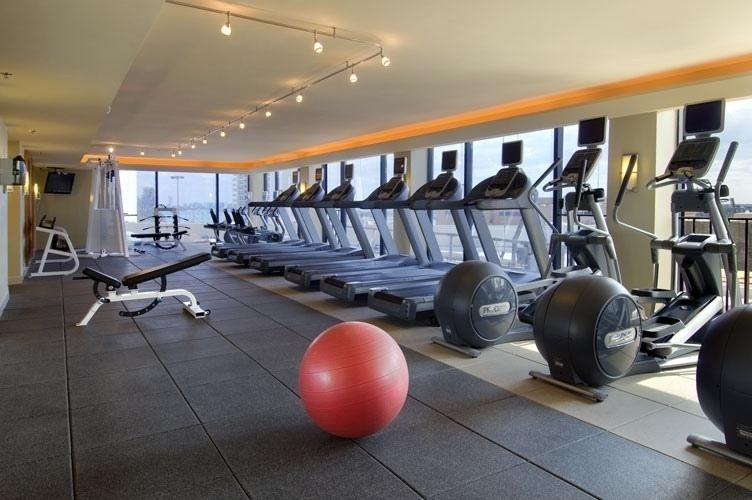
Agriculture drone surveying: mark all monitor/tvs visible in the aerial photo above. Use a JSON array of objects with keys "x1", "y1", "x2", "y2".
[
  {"x1": 248, "y1": 191, "x2": 252, "y2": 202},
  {"x1": 393, "y1": 156, "x2": 408, "y2": 175},
  {"x1": 683, "y1": 97, "x2": 726, "y2": 136},
  {"x1": 344, "y1": 164, "x2": 355, "y2": 179},
  {"x1": 44, "y1": 172, "x2": 76, "y2": 194},
  {"x1": 501, "y1": 140, "x2": 523, "y2": 166},
  {"x1": 272, "y1": 191, "x2": 278, "y2": 200},
  {"x1": 315, "y1": 168, "x2": 324, "y2": 183},
  {"x1": 292, "y1": 171, "x2": 300, "y2": 184},
  {"x1": 261, "y1": 191, "x2": 268, "y2": 200},
  {"x1": 441, "y1": 150, "x2": 458, "y2": 172},
  {"x1": 577, "y1": 116, "x2": 608, "y2": 147}
]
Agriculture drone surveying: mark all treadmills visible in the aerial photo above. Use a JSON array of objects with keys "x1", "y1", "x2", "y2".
[
  {"x1": 319, "y1": 149, "x2": 481, "y2": 307},
  {"x1": 283, "y1": 156, "x2": 432, "y2": 294},
  {"x1": 366, "y1": 140, "x2": 553, "y2": 328},
  {"x1": 211, "y1": 163, "x2": 375, "y2": 275}
]
